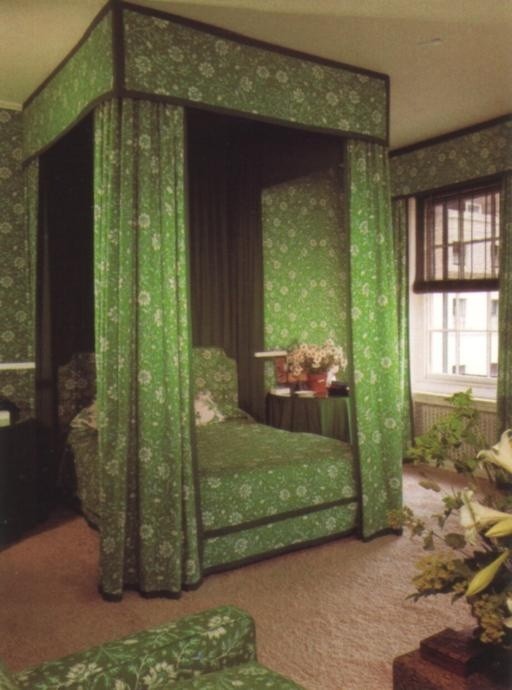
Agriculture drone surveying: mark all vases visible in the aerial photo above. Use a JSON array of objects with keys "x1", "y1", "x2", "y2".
[{"x1": 307, "y1": 369, "x2": 329, "y2": 391}]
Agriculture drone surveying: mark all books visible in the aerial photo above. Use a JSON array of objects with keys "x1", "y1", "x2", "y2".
[{"x1": 266, "y1": 380, "x2": 350, "y2": 401}]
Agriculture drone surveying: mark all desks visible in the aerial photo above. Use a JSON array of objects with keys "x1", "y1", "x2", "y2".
[{"x1": 268, "y1": 386, "x2": 353, "y2": 444}]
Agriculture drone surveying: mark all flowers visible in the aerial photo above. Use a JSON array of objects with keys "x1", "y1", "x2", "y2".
[
  {"x1": 379, "y1": 388, "x2": 511, "y2": 651},
  {"x1": 290, "y1": 337, "x2": 347, "y2": 383}
]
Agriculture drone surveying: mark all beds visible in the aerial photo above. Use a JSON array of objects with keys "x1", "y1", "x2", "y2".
[{"x1": 59, "y1": 345, "x2": 359, "y2": 573}]
